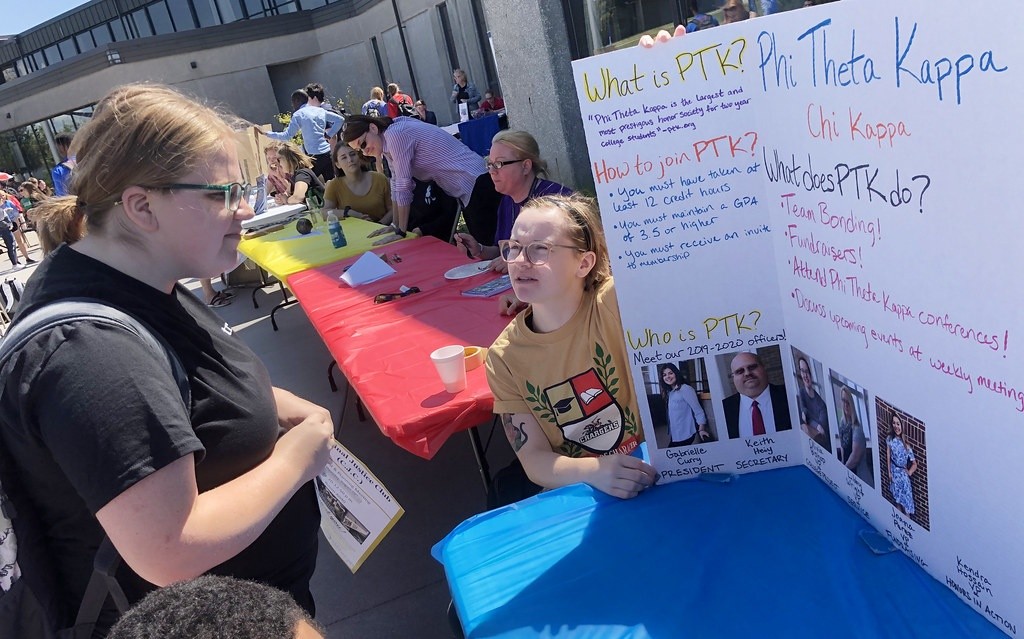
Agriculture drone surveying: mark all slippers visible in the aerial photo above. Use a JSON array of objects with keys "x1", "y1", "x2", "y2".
[
  {"x1": 206, "y1": 294, "x2": 231, "y2": 308},
  {"x1": 216, "y1": 291, "x2": 236, "y2": 299}
]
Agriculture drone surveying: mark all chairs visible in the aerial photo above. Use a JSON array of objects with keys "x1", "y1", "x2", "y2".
[{"x1": 458, "y1": 114, "x2": 501, "y2": 157}]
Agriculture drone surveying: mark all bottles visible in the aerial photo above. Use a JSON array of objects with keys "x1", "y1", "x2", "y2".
[{"x1": 326, "y1": 210, "x2": 347, "y2": 249}]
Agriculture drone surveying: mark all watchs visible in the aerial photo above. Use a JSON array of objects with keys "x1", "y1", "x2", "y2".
[{"x1": 395, "y1": 228, "x2": 407, "y2": 238}]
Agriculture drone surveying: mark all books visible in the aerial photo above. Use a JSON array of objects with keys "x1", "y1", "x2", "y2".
[{"x1": 461, "y1": 275, "x2": 512, "y2": 298}]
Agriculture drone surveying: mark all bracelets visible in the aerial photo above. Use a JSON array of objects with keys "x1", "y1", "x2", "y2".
[
  {"x1": 391, "y1": 223, "x2": 397, "y2": 229},
  {"x1": 343, "y1": 206, "x2": 351, "y2": 217},
  {"x1": 698, "y1": 430, "x2": 704, "y2": 432},
  {"x1": 473, "y1": 243, "x2": 483, "y2": 257},
  {"x1": 286, "y1": 196, "x2": 290, "y2": 204}
]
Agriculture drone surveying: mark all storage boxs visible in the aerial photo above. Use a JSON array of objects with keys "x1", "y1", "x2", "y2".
[{"x1": 227, "y1": 258, "x2": 267, "y2": 285}]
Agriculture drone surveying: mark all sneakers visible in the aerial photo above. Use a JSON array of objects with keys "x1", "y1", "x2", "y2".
[
  {"x1": 25, "y1": 259, "x2": 42, "y2": 268},
  {"x1": 12, "y1": 261, "x2": 26, "y2": 272}
]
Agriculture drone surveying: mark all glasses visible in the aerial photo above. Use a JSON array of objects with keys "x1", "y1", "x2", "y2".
[
  {"x1": 453, "y1": 75, "x2": 461, "y2": 79},
  {"x1": 415, "y1": 104, "x2": 423, "y2": 108},
  {"x1": 484, "y1": 159, "x2": 524, "y2": 170},
  {"x1": 114, "y1": 181, "x2": 251, "y2": 212},
  {"x1": 277, "y1": 157, "x2": 282, "y2": 163},
  {"x1": 19, "y1": 189, "x2": 24, "y2": 193},
  {"x1": 729, "y1": 362, "x2": 761, "y2": 375},
  {"x1": 486, "y1": 96, "x2": 493, "y2": 100},
  {"x1": 498, "y1": 240, "x2": 587, "y2": 266},
  {"x1": 356, "y1": 132, "x2": 367, "y2": 157},
  {"x1": 841, "y1": 398, "x2": 850, "y2": 404},
  {"x1": 799, "y1": 368, "x2": 810, "y2": 373}
]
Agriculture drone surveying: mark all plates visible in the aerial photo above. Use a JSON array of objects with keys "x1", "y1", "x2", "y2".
[{"x1": 444, "y1": 259, "x2": 493, "y2": 280}]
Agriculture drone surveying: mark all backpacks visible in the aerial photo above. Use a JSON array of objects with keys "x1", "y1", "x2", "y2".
[
  {"x1": 366, "y1": 101, "x2": 381, "y2": 119},
  {"x1": 1, "y1": 300, "x2": 192, "y2": 639},
  {"x1": 390, "y1": 94, "x2": 421, "y2": 122}
]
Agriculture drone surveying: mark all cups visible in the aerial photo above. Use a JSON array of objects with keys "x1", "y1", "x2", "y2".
[{"x1": 429, "y1": 344, "x2": 466, "y2": 394}]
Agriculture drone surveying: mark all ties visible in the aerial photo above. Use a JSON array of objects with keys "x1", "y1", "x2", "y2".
[{"x1": 751, "y1": 400, "x2": 766, "y2": 436}]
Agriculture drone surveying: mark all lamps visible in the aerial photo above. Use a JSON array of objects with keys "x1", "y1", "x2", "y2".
[
  {"x1": 106, "y1": 50, "x2": 122, "y2": 66},
  {"x1": 6, "y1": 112, "x2": 10, "y2": 118},
  {"x1": 190, "y1": 62, "x2": 197, "y2": 69}
]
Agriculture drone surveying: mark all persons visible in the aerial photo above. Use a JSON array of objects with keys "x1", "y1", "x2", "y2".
[
  {"x1": 200, "y1": 69, "x2": 573, "y2": 318},
  {"x1": 659, "y1": 362, "x2": 709, "y2": 445},
  {"x1": 686, "y1": 0, "x2": 815, "y2": 34},
  {"x1": 105, "y1": 576, "x2": 332, "y2": 638},
  {"x1": 722, "y1": 352, "x2": 792, "y2": 439},
  {"x1": 798, "y1": 357, "x2": 832, "y2": 454},
  {"x1": 0, "y1": 131, "x2": 77, "y2": 271},
  {"x1": 839, "y1": 386, "x2": 875, "y2": 488},
  {"x1": 886, "y1": 413, "x2": 917, "y2": 518},
  {"x1": 0, "y1": 83, "x2": 332, "y2": 639},
  {"x1": 485, "y1": 25, "x2": 685, "y2": 500}
]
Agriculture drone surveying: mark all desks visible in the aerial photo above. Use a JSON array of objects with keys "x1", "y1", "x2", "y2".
[
  {"x1": 441, "y1": 109, "x2": 506, "y2": 138},
  {"x1": 237, "y1": 212, "x2": 418, "y2": 329},
  {"x1": 428, "y1": 440, "x2": 1011, "y2": 638},
  {"x1": 288, "y1": 238, "x2": 530, "y2": 511}
]
